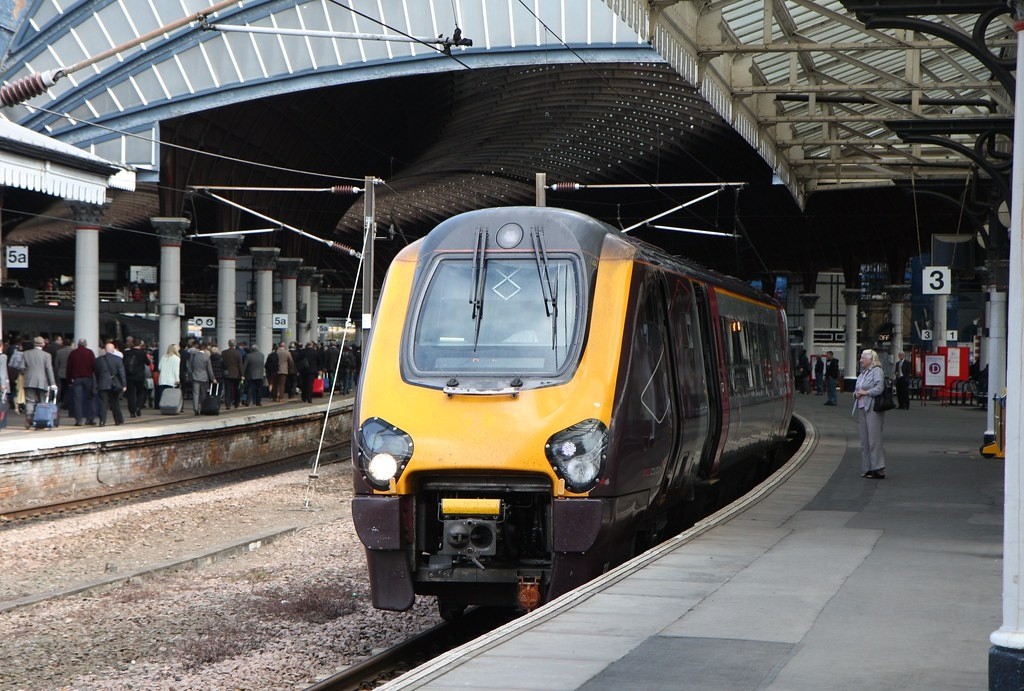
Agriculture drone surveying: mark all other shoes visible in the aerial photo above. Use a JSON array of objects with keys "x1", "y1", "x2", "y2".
[
  {"x1": 195, "y1": 412, "x2": 199, "y2": 416},
  {"x1": 116, "y1": 420, "x2": 123, "y2": 425},
  {"x1": 141, "y1": 383, "x2": 353, "y2": 410},
  {"x1": 100, "y1": 421, "x2": 104, "y2": 427},
  {"x1": 895, "y1": 405, "x2": 909, "y2": 410},
  {"x1": 800, "y1": 390, "x2": 825, "y2": 396},
  {"x1": 824, "y1": 400, "x2": 835, "y2": 405},
  {"x1": 130, "y1": 411, "x2": 141, "y2": 417},
  {"x1": 25, "y1": 416, "x2": 31, "y2": 430},
  {"x1": 75, "y1": 422, "x2": 81, "y2": 426},
  {"x1": 85, "y1": 421, "x2": 96, "y2": 425}
]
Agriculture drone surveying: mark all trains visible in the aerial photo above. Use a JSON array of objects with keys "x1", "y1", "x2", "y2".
[
  {"x1": 349, "y1": 205, "x2": 795, "y2": 621},
  {"x1": 0, "y1": 279, "x2": 158, "y2": 351}
]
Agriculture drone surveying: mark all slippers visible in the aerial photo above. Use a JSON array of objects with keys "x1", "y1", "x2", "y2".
[
  {"x1": 866, "y1": 472, "x2": 885, "y2": 479},
  {"x1": 860, "y1": 471, "x2": 872, "y2": 477}
]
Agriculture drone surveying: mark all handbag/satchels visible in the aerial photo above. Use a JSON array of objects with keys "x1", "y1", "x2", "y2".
[
  {"x1": 873, "y1": 379, "x2": 897, "y2": 411},
  {"x1": 111, "y1": 375, "x2": 123, "y2": 393}
]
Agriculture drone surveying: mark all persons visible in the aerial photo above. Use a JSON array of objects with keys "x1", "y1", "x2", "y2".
[
  {"x1": 507, "y1": 309, "x2": 568, "y2": 348},
  {"x1": 796, "y1": 350, "x2": 810, "y2": 396},
  {"x1": 815, "y1": 352, "x2": 825, "y2": 396},
  {"x1": 1, "y1": 266, "x2": 363, "y2": 431},
  {"x1": 851, "y1": 349, "x2": 886, "y2": 480},
  {"x1": 821, "y1": 350, "x2": 839, "y2": 407},
  {"x1": 895, "y1": 351, "x2": 912, "y2": 410}
]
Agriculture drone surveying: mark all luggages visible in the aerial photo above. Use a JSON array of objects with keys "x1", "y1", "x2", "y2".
[
  {"x1": 311, "y1": 374, "x2": 324, "y2": 397},
  {"x1": 32, "y1": 386, "x2": 58, "y2": 430},
  {"x1": 0, "y1": 388, "x2": 9, "y2": 428},
  {"x1": 159, "y1": 383, "x2": 183, "y2": 414},
  {"x1": 322, "y1": 372, "x2": 329, "y2": 391},
  {"x1": 201, "y1": 382, "x2": 221, "y2": 415}
]
